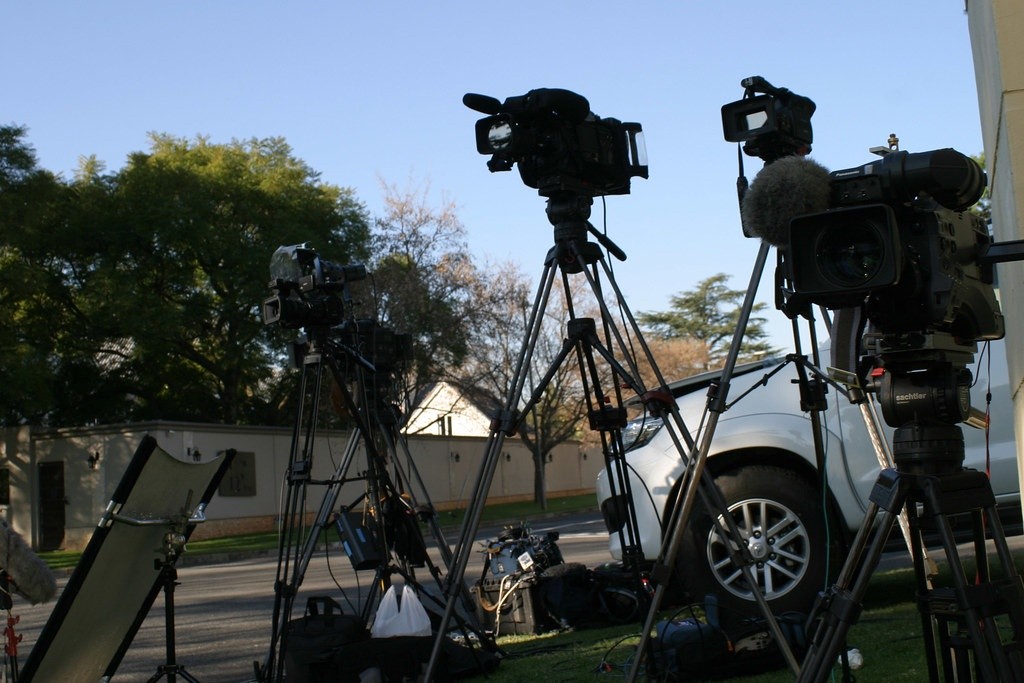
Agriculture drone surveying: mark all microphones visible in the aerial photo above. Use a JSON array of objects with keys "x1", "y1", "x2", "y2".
[
  {"x1": 463, "y1": 93, "x2": 505, "y2": 115},
  {"x1": 739, "y1": 154, "x2": 833, "y2": 250}
]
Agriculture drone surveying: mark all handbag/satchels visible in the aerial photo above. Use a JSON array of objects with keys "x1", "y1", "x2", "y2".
[
  {"x1": 371, "y1": 584, "x2": 433, "y2": 639},
  {"x1": 285, "y1": 595, "x2": 367, "y2": 680}
]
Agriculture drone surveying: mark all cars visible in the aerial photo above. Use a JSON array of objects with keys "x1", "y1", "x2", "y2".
[{"x1": 599, "y1": 226, "x2": 1019, "y2": 618}]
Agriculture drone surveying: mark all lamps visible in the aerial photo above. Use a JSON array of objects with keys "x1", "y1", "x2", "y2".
[
  {"x1": 503, "y1": 451, "x2": 511, "y2": 462},
  {"x1": 451, "y1": 451, "x2": 460, "y2": 462},
  {"x1": 187, "y1": 447, "x2": 201, "y2": 461},
  {"x1": 579, "y1": 451, "x2": 587, "y2": 461},
  {"x1": 546, "y1": 452, "x2": 553, "y2": 461},
  {"x1": 87, "y1": 451, "x2": 99, "y2": 468}
]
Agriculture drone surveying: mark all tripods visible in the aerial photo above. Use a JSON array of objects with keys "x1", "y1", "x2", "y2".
[{"x1": 262, "y1": 197, "x2": 1024, "y2": 682}]
